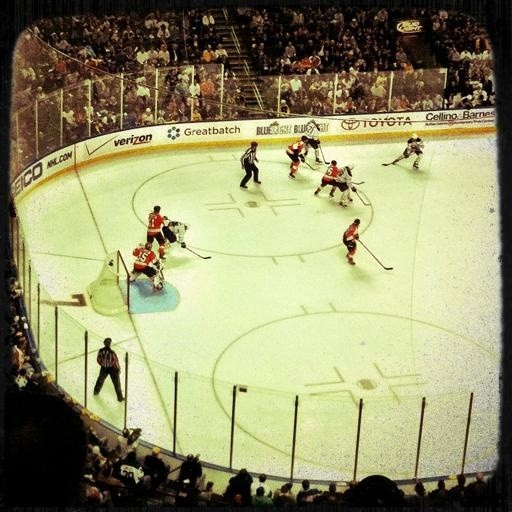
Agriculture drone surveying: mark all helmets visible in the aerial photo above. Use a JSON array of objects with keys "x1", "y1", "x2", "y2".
[
  {"x1": 251, "y1": 141, "x2": 258, "y2": 146},
  {"x1": 331, "y1": 160, "x2": 336, "y2": 165}
]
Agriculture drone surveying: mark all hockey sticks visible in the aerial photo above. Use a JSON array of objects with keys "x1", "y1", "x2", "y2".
[
  {"x1": 305, "y1": 162, "x2": 321, "y2": 171},
  {"x1": 356, "y1": 239, "x2": 394, "y2": 270},
  {"x1": 352, "y1": 182, "x2": 365, "y2": 185},
  {"x1": 356, "y1": 192, "x2": 372, "y2": 206},
  {"x1": 318, "y1": 144, "x2": 330, "y2": 164},
  {"x1": 177, "y1": 241, "x2": 212, "y2": 259},
  {"x1": 382, "y1": 152, "x2": 413, "y2": 166}
]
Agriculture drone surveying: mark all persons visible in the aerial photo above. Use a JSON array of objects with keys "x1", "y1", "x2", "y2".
[
  {"x1": 239, "y1": 141, "x2": 261, "y2": 189},
  {"x1": 342, "y1": 218, "x2": 360, "y2": 264},
  {"x1": 162, "y1": 221, "x2": 188, "y2": 249},
  {"x1": 146, "y1": 206, "x2": 166, "y2": 260},
  {"x1": 129, "y1": 243, "x2": 163, "y2": 290},
  {"x1": 337, "y1": 167, "x2": 357, "y2": 207},
  {"x1": 315, "y1": 159, "x2": 341, "y2": 197}
]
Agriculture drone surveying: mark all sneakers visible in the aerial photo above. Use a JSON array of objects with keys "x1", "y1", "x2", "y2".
[
  {"x1": 254, "y1": 180, "x2": 261, "y2": 184},
  {"x1": 289, "y1": 173, "x2": 295, "y2": 178},
  {"x1": 330, "y1": 193, "x2": 334, "y2": 197},
  {"x1": 315, "y1": 189, "x2": 319, "y2": 195},
  {"x1": 240, "y1": 184, "x2": 248, "y2": 189},
  {"x1": 315, "y1": 158, "x2": 322, "y2": 163}
]
